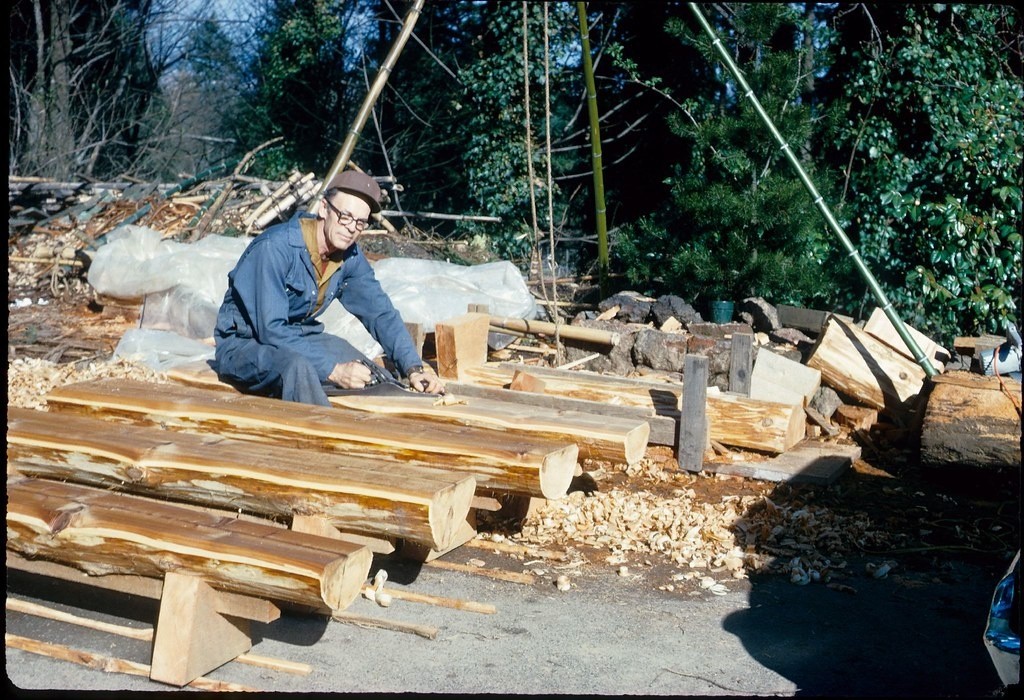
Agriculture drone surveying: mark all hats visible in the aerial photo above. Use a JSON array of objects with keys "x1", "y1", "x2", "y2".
[{"x1": 327, "y1": 170, "x2": 382, "y2": 213}]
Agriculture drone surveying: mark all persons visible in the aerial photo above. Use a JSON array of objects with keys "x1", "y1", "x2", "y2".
[{"x1": 214, "y1": 171, "x2": 444, "y2": 406}]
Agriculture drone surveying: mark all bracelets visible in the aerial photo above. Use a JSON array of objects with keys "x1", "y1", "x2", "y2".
[{"x1": 407, "y1": 366, "x2": 423, "y2": 379}]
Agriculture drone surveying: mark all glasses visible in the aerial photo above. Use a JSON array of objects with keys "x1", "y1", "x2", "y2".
[{"x1": 324, "y1": 196, "x2": 369, "y2": 231}]
